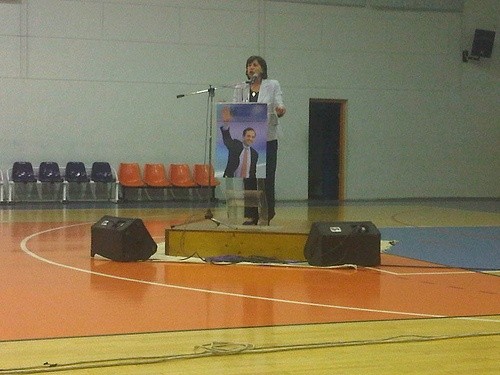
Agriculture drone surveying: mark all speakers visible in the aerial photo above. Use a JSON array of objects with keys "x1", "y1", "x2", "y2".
[
  {"x1": 91, "y1": 215, "x2": 158, "y2": 262},
  {"x1": 471, "y1": 29, "x2": 496, "y2": 58},
  {"x1": 303, "y1": 221, "x2": 381, "y2": 267}
]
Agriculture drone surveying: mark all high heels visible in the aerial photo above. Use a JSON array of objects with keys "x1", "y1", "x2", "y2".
[{"x1": 242, "y1": 216, "x2": 259, "y2": 225}]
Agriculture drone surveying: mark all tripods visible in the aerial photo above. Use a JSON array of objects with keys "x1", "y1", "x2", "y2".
[{"x1": 169, "y1": 81, "x2": 252, "y2": 229}]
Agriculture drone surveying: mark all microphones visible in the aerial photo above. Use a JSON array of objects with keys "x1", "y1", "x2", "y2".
[{"x1": 251, "y1": 74, "x2": 259, "y2": 84}]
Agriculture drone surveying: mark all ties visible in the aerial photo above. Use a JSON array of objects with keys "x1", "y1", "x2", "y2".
[{"x1": 239, "y1": 147, "x2": 249, "y2": 178}]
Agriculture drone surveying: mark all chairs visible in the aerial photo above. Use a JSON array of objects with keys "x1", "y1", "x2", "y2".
[{"x1": 6, "y1": 159, "x2": 220, "y2": 204}]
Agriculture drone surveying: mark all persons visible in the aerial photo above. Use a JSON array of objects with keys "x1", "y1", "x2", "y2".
[
  {"x1": 232, "y1": 56, "x2": 286, "y2": 225},
  {"x1": 220, "y1": 105, "x2": 259, "y2": 180}
]
